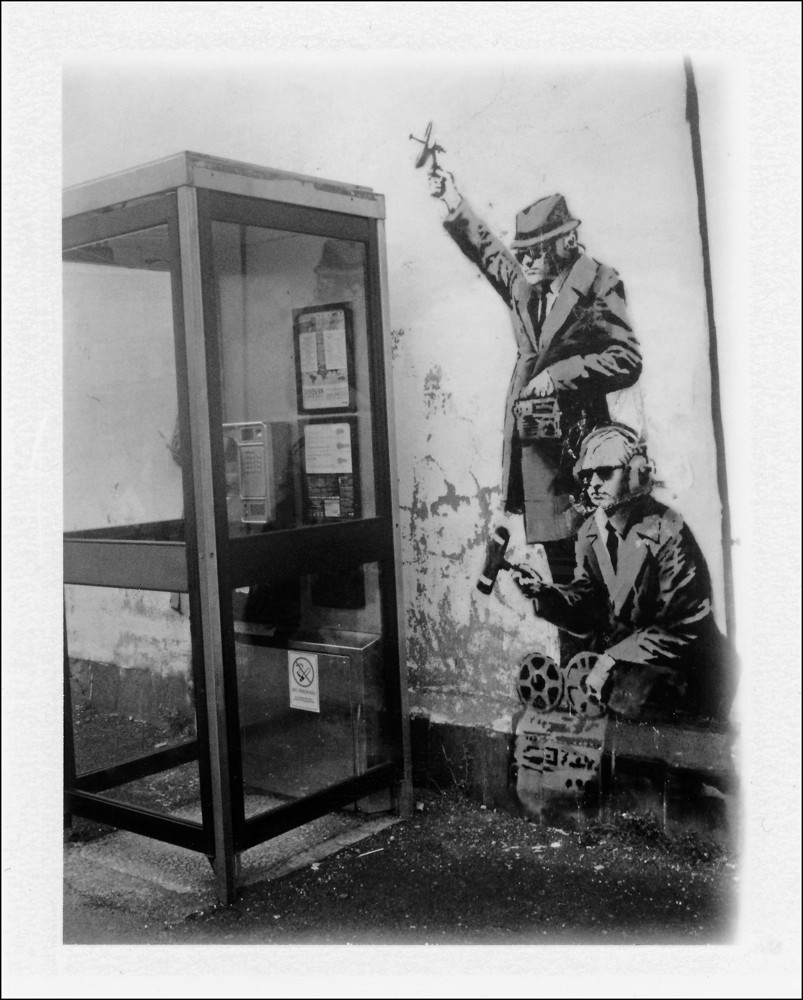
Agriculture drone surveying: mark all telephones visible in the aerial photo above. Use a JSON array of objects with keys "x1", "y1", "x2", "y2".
[{"x1": 221, "y1": 420, "x2": 290, "y2": 525}]
